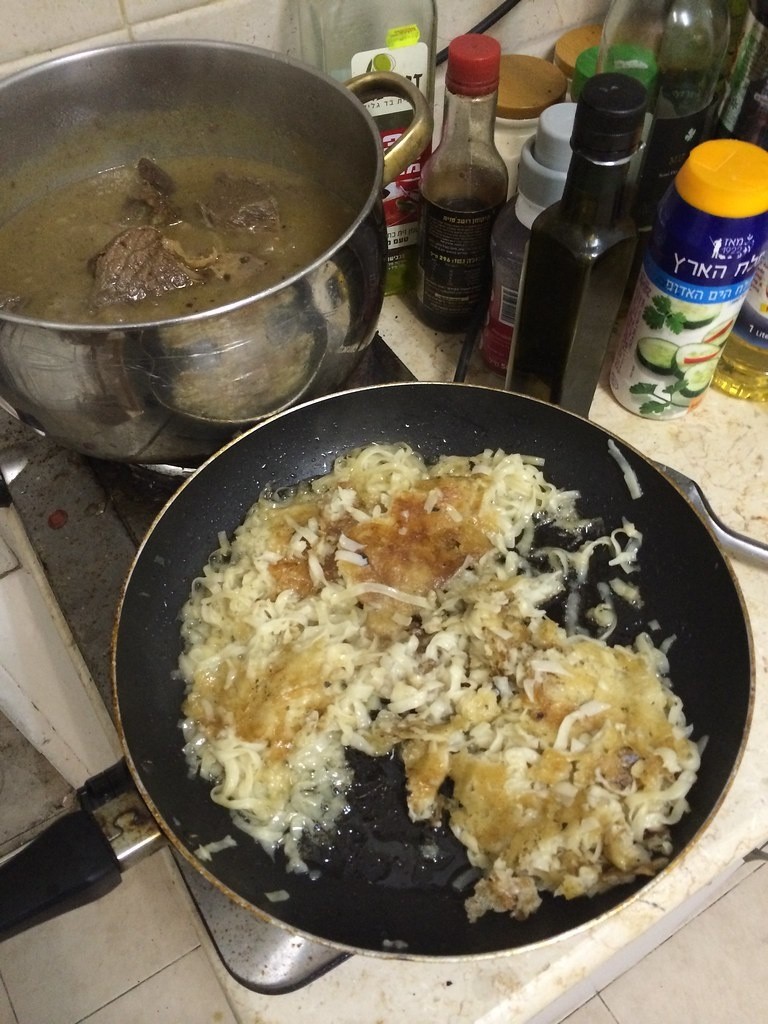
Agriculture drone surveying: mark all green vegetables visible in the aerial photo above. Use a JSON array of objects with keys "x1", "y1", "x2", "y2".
[
  {"x1": 629, "y1": 380, "x2": 690, "y2": 414},
  {"x1": 641, "y1": 295, "x2": 687, "y2": 336}
]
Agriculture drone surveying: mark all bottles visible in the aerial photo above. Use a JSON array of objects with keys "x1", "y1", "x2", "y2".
[
  {"x1": 595, "y1": 0, "x2": 731, "y2": 298},
  {"x1": 606, "y1": 138, "x2": 768, "y2": 420},
  {"x1": 417, "y1": 34, "x2": 508, "y2": 333},
  {"x1": 478, "y1": 102, "x2": 581, "y2": 377},
  {"x1": 295, "y1": 0, "x2": 437, "y2": 297},
  {"x1": 493, "y1": 24, "x2": 658, "y2": 200},
  {"x1": 713, "y1": 0, "x2": 768, "y2": 150},
  {"x1": 501, "y1": 73, "x2": 647, "y2": 420}
]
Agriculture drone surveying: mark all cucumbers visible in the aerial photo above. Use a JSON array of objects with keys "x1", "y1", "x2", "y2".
[{"x1": 636, "y1": 295, "x2": 740, "y2": 399}]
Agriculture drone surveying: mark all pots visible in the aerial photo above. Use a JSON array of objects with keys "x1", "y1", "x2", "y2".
[
  {"x1": 0, "y1": 382, "x2": 754, "y2": 974},
  {"x1": 0, "y1": 37, "x2": 434, "y2": 463}
]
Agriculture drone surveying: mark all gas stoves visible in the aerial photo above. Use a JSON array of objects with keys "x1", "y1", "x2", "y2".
[{"x1": 0, "y1": 332, "x2": 645, "y2": 995}]
